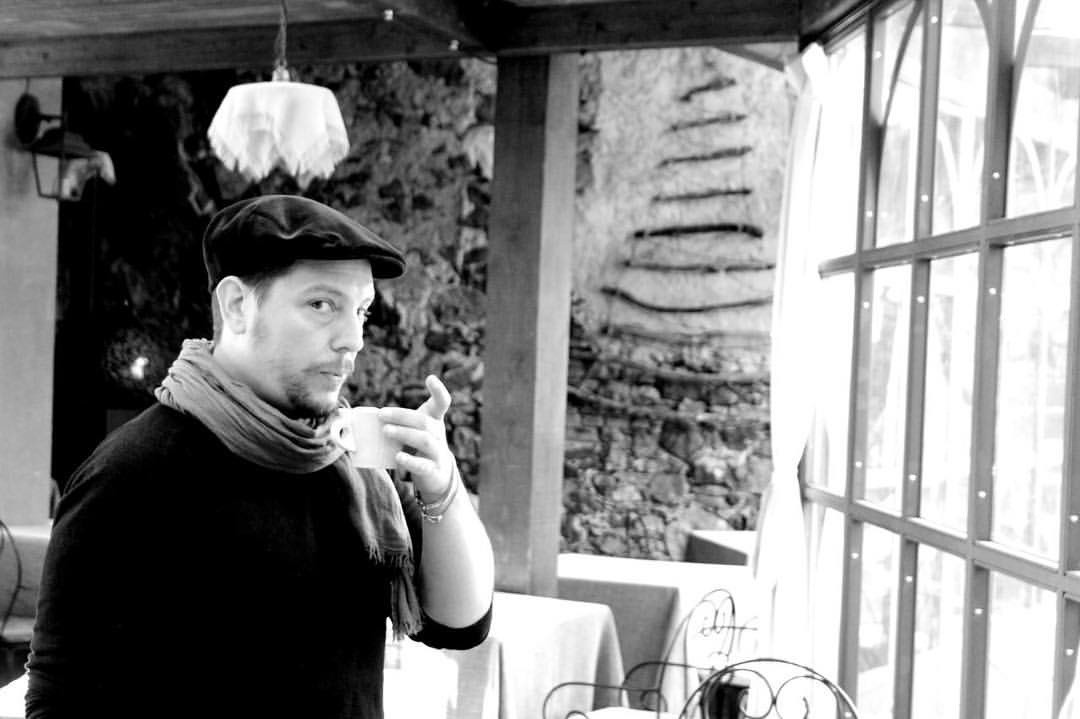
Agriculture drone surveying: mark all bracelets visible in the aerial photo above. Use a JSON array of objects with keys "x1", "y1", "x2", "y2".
[{"x1": 412, "y1": 451, "x2": 460, "y2": 524}]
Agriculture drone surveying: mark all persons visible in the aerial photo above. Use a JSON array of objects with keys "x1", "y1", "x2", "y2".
[{"x1": 24, "y1": 193, "x2": 498, "y2": 719}]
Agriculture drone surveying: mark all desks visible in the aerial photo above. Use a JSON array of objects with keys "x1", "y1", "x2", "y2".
[
  {"x1": 558, "y1": 547, "x2": 751, "y2": 711},
  {"x1": 687, "y1": 528, "x2": 758, "y2": 566},
  {"x1": 381, "y1": 589, "x2": 627, "y2": 719}
]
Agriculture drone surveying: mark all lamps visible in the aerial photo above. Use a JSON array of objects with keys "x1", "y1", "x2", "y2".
[
  {"x1": 209, "y1": 0, "x2": 354, "y2": 188},
  {"x1": 13, "y1": 91, "x2": 96, "y2": 199}
]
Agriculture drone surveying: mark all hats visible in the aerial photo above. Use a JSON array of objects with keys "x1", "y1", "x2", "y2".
[{"x1": 202, "y1": 194, "x2": 406, "y2": 296}]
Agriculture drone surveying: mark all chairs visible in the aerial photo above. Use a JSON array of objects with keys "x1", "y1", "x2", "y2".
[{"x1": 543, "y1": 587, "x2": 861, "y2": 717}]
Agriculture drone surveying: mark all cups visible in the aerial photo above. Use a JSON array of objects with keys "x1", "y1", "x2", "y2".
[{"x1": 329, "y1": 407, "x2": 403, "y2": 469}]
